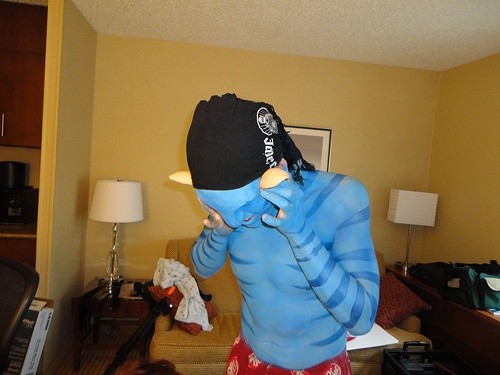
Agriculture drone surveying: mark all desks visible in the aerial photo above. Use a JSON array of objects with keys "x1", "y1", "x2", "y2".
[{"x1": 71, "y1": 279, "x2": 154, "y2": 372}]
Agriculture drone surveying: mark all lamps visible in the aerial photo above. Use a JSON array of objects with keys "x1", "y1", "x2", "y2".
[
  {"x1": 89, "y1": 179, "x2": 144, "y2": 294},
  {"x1": 387, "y1": 189, "x2": 439, "y2": 270}
]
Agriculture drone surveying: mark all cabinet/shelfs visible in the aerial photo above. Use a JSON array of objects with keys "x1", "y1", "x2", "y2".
[
  {"x1": 388, "y1": 265, "x2": 500, "y2": 375},
  {"x1": 0, "y1": 233, "x2": 36, "y2": 271},
  {"x1": 0, "y1": 0, "x2": 48, "y2": 149}
]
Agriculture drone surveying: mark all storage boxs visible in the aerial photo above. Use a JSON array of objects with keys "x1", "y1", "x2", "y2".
[
  {"x1": 383, "y1": 342, "x2": 484, "y2": 375},
  {"x1": 0, "y1": 297, "x2": 55, "y2": 375}
]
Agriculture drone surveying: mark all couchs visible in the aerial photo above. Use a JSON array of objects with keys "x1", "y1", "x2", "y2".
[{"x1": 149, "y1": 239, "x2": 432, "y2": 375}]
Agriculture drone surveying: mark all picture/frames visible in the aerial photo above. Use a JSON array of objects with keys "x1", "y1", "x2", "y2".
[{"x1": 283, "y1": 125, "x2": 332, "y2": 172}]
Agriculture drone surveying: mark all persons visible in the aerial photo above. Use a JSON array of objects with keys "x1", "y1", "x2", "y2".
[{"x1": 185, "y1": 92, "x2": 380, "y2": 375}]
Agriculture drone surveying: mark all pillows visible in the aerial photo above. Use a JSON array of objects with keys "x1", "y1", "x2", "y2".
[
  {"x1": 147, "y1": 286, "x2": 217, "y2": 336},
  {"x1": 375, "y1": 271, "x2": 433, "y2": 330}
]
